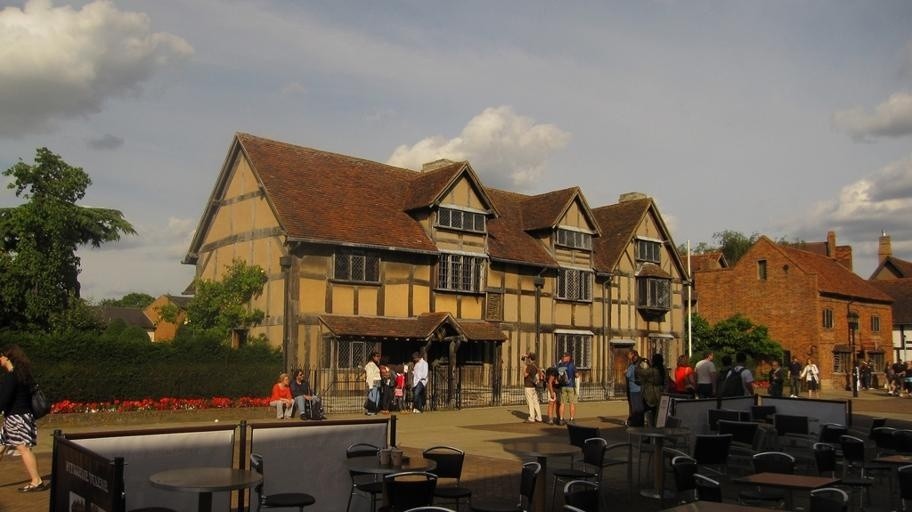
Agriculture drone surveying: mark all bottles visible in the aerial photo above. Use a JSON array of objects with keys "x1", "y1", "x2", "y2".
[{"x1": 376, "y1": 448, "x2": 380, "y2": 463}]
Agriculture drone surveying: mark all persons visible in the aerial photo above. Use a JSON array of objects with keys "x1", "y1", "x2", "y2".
[
  {"x1": 523, "y1": 352, "x2": 580, "y2": 427},
  {"x1": 767, "y1": 357, "x2": 820, "y2": 400},
  {"x1": 623, "y1": 350, "x2": 754, "y2": 426},
  {"x1": 0, "y1": 344, "x2": 49, "y2": 494},
  {"x1": 289, "y1": 369, "x2": 327, "y2": 420},
  {"x1": 855, "y1": 359, "x2": 912, "y2": 397},
  {"x1": 270, "y1": 373, "x2": 295, "y2": 420},
  {"x1": 363, "y1": 350, "x2": 430, "y2": 416}
]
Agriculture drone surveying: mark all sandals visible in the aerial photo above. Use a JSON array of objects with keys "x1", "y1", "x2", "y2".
[{"x1": 18, "y1": 482, "x2": 44, "y2": 492}]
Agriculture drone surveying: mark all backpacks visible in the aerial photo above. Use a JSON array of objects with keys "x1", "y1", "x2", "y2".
[
  {"x1": 534, "y1": 368, "x2": 545, "y2": 388},
  {"x1": 724, "y1": 368, "x2": 744, "y2": 396}
]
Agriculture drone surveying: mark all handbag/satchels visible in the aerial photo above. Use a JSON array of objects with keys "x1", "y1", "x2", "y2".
[
  {"x1": 395, "y1": 389, "x2": 403, "y2": 396},
  {"x1": 305, "y1": 397, "x2": 324, "y2": 419},
  {"x1": 685, "y1": 376, "x2": 697, "y2": 394},
  {"x1": 364, "y1": 399, "x2": 376, "y2": 410},
  {"x1": 31, "y1": 383, "x2": 50, "y2": 419}
]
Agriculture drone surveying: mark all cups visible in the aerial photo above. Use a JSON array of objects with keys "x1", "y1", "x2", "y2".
[
  {"x1": 391, "y1": 449, "x2": 403, "y2": 466},
  {"x1": 380, "y1": 448, "x2": 391, "y2": 464}
]
